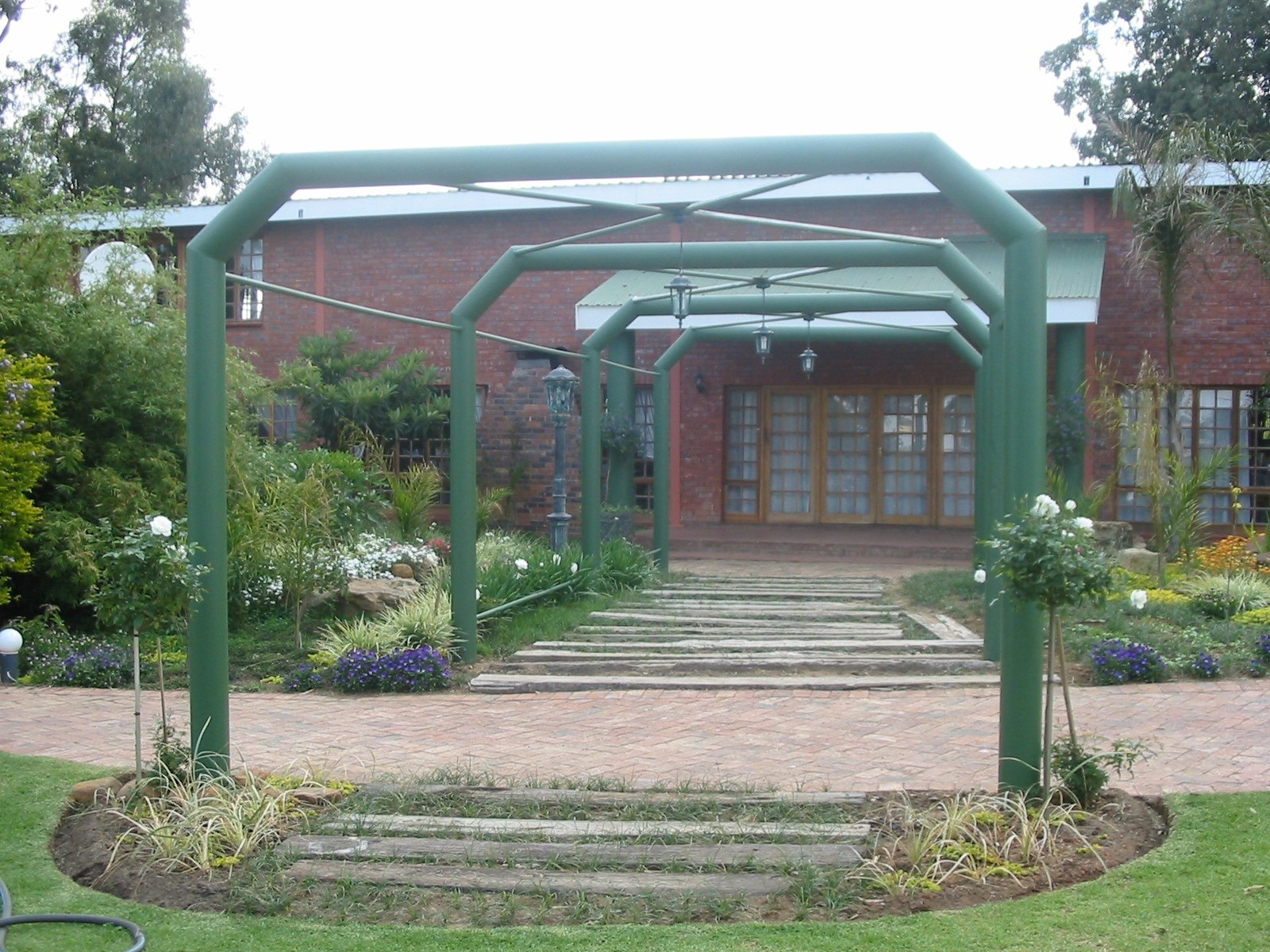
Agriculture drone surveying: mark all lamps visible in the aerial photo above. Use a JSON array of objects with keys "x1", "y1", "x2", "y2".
[
  {"x1": 799, "y1": 315, "x2": 817, "y2": 379},
  {"x1": 0, "y1": 628, "x2": 23, "y2": 654},
  {"x1": 752, "y1": 282, "x2": 774, "y2": 361},
  {"x1": 663, "y1": 211, "x2": 698, "y2": 333},
  {"x1": 694, "y1": 373, "x2": 705, "y2": 393}
]
